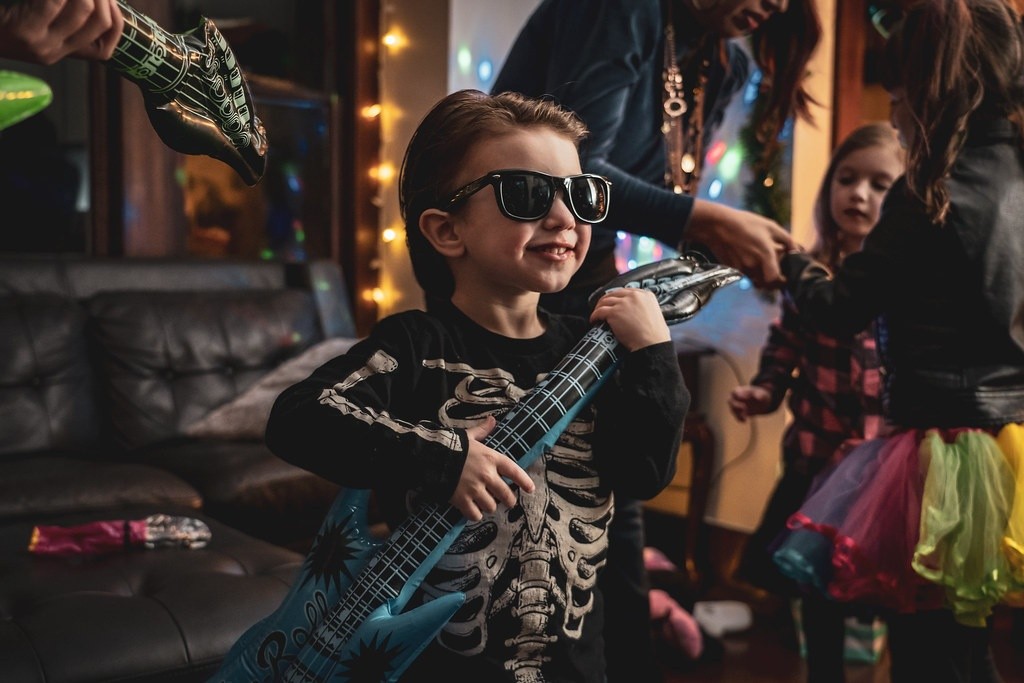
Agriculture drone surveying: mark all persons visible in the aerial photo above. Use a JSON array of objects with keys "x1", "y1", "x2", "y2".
[
  {"x1": 0, "y1": 0, "x2": 124, "y2": 66},
  {"x1": 265, "y1": 0, "x2": 1023, "y2": 683}
]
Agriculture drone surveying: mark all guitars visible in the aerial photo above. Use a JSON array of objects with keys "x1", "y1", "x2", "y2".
[
  {"x1": 96, "y1": 0, "x2": 269, "y2": 190},
  {"x1": 195, "y1": 254, "x2": 744, "y2": 682}
]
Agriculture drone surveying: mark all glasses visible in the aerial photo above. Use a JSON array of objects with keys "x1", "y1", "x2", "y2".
[{"x1": 441, "y1": 173, "x2": 611, "y2": 229}]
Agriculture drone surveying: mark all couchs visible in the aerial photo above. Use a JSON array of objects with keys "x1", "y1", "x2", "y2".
[{"x1": 1, "y1": 257, "x2": 361, "y2": 682}]
